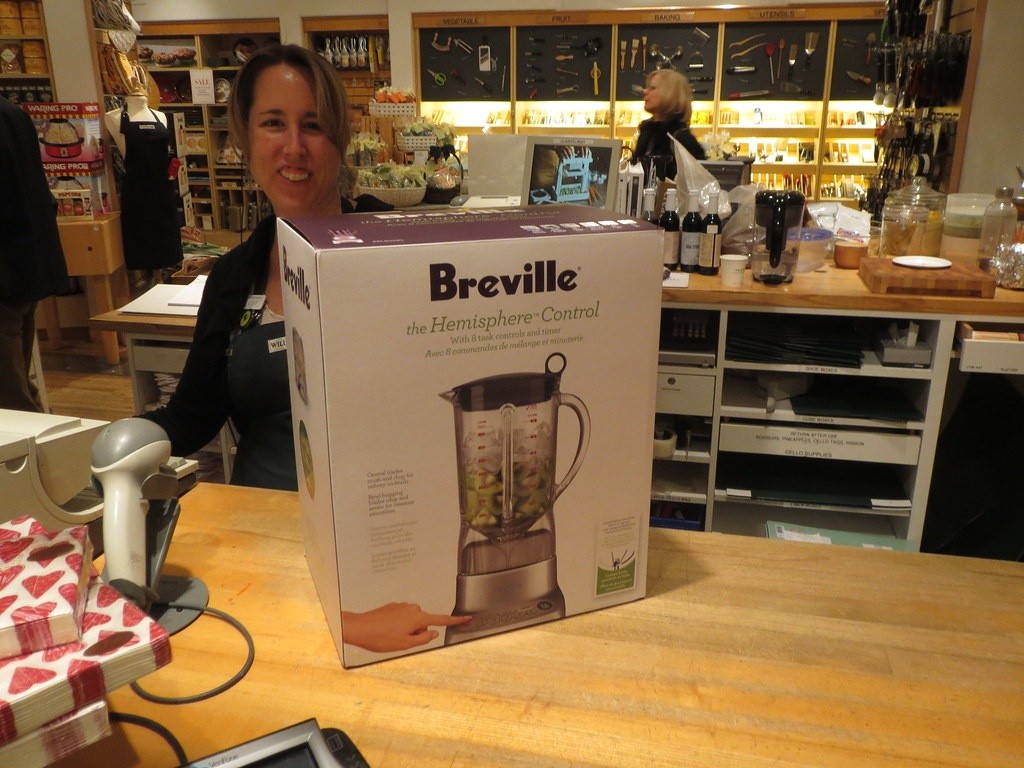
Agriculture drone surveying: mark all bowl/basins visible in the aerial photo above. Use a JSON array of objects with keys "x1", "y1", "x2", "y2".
[
  {"x1": 137, "y1": 47, "x2": 195, "y2": 67},
  {"x1": 943, "y1": 192, "x2": 996, "y2": 238},
  {"x1": 786, "y1": 227, "x2": 833, "y2": 272},
  {"x1": 825, "y1": 226, "x2": 881, "y2": 270}
]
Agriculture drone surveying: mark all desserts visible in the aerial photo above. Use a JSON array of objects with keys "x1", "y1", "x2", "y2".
[{"x1": 137, "y1": 47, "x2": 196, "y2": 67}]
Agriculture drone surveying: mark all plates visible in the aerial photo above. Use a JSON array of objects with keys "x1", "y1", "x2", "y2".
[
  {"x1": 214, "y1": 75, "x2": 235, "y2": 103},
  {"x1": 891, "y1": 255, "x2": 953, "y2": 269},
  {"x1": 231, "y1": 37, "x2": 260, "y2": 65},
  {"x1": 151, "y1": 73, "x2": 192, "y2": 103}
]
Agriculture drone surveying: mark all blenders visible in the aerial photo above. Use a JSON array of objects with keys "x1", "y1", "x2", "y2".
[{"x1": 438, "y1": 352, "x2": 591, "y2": 646}]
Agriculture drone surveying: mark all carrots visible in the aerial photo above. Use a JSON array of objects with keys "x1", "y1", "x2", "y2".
[{"x1": 375, "y1": 91, "x2": 408, "y2": 104}]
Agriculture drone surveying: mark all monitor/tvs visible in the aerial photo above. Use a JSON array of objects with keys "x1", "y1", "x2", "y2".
[{"x1": 462, "y1": 133, "x2": 623, "y2": 212}]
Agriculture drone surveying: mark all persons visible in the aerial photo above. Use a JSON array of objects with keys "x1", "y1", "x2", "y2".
[
  {"x1": 134, "y1": 45, "x2": 394, "y2": 493},
  {"x1": 0, "y1": 95, "x2": 71, "y2": 411},
  {"x1": 632, "y1": 69, "x2": 705, "y2": 182},
  {"x1": 106, "y1": 96, "x2": 184, "y2": 270}
]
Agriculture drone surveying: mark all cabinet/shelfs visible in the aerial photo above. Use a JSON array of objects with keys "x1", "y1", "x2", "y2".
[
  {"x1": 648, "y1": 305, "x2": 959, "y2": 544},
  {"x1": 0, "y1": 0, "x2": 58, "y2": 108},
  {"x1": 94, "y1": 21, "x2": 389, "y2": 231},
  {"x1": 421, "y1": 99, "x2": 886, "y2": 198}
]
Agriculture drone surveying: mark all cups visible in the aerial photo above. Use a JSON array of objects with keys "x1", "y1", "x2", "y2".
[{"x1": 720, "y1": 254, "x2": 748, "y2": 288}]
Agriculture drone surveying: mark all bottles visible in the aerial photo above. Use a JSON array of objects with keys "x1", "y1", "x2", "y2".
[
  {"x1": 977, "y1": 185, "x2": 1019, "y2": 272},
  {"x1": 877, "y1": 177, "x2": 947, "y2": 258},
  {"x1": 680, "y1": 190, "x2": 703, "y2": 273},
  {"x1": 660, "y1": 189, "x2": 680, "y2": 271},
  {"x1": 317, "y1": 34, "x2": 369, "y2": 69},
  {"x1": 640, "y1": 187, "x2": 659, "y2": 227},
  {"x1": 697, "y1": 192, "x2": 722, "y2": 275}
]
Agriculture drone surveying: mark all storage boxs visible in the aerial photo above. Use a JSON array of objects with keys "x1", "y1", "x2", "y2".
[
  {"x1": 276, "y1": 206, "x2": 665, "y2": 674},
  {"x1": 369, "y1": 104, "x2": 439, "y2": 151}
]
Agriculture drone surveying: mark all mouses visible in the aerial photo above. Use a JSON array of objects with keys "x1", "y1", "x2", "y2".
[{"x1": 449, "y1": 194, "x2": 469, "y2": 205}]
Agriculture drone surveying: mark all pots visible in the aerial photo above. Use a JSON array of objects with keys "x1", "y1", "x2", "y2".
[{"x1": 39, "y1": 117, "x2": 84, "y2": 158}]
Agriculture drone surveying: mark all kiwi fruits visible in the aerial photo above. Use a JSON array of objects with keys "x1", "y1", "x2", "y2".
[{"x1": 462, "y1": 461, "x2": 546, "y2": 528}]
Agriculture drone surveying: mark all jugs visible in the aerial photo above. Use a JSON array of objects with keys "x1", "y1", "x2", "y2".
[{"x1": 751, "y1": 189, "x2": 807, "y2": 284}]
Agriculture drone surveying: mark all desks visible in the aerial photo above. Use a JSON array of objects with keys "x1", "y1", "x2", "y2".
[{"x1": 53, "y1": 481, "x2": 1024, "y2": 768}]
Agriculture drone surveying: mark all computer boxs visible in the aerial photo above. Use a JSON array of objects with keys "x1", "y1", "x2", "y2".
[{"x1": 625, "y1": 160, "x2": 645, "y2": 219}]
